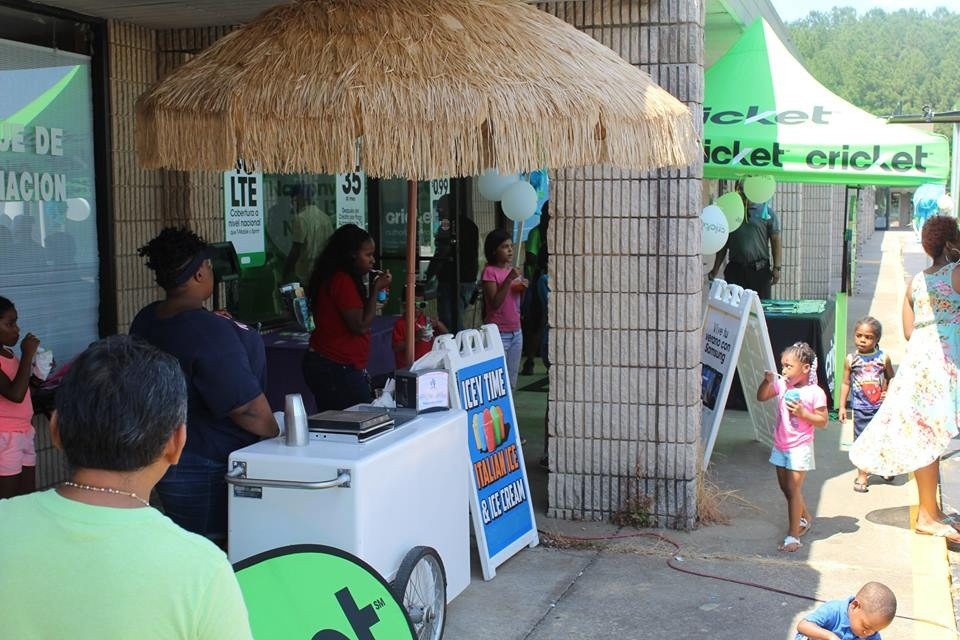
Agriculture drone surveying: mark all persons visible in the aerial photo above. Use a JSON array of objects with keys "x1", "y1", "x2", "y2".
[
  {"x1": 0, "y1": 333, "x2": 259, "y2": 640},
  {"x1": 838, "y1": 316, "x2": 896, "y2": 493},
  {"x1": 849, "y1": 214, "x2": 960, "y2": 544},
  {"x1": 392, "y1": 283, "x2": 451, "y2": 370},
  {"x1": 0, "y1": 295, "x2": 59, "y2": 500},
  {"x1": 127, "y1": 224, "x2": 285, "y2": 555},
  {"x1": 792, "y1": 582, "x2": 897, "y2": 640},
  {"x1": 708, "y1": 180, "x2": 782, "y2": 300},
  {"x1": 305, "y1": 222, "x2": 392, "y2": 414},
  {"x1": 286, "y1": 182, "x2": 335, "y2": 323},
  {"x1": 482, "y1": 200, "x2": 549, "y2": 470},
  {"x1": 416, "y1": 194, "x2": 480, "y2": 339},
  {"x1": 757, "y1": 340, "x2": 829, "y2": 552}
]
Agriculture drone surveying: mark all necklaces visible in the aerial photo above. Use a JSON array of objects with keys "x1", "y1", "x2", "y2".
[{"x1": 58, "y1": 478, "x2": 151, "y2": 509}]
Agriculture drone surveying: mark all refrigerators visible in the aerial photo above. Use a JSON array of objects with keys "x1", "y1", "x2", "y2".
[{"x1": 227, "y1": 410, "x2": 471, "y2": 634}]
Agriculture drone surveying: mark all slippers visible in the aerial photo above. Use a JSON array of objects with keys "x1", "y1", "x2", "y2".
[
  {"x1": 777, "y1": 536, "x2": 803, "y2": 553},
  {"x1": 854, "y1": 478, "x2": 868, "y2": 492},
  {"x1": 799, "y1": 517, "x2": 812, "y2": 536},
  {"x1": 880, "y1": 476, "x2": 895, "y2": 482}
]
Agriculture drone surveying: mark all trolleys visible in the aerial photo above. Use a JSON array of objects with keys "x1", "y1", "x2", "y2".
[{"x1": 225, "y1": 401, "x2": 471, "y2": 640}]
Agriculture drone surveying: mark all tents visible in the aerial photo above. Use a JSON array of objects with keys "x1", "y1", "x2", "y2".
[{"x1": 699, "y1": 14, "x2": 951, "y2": 199}]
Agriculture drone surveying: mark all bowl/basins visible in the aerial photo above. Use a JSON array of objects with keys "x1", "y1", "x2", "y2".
[
  {"x1": 785, "y1": 396, "x2": 800, "y2": 406},
  {"x1": 377, "y1": 289, "x2": 386, "y2": 301}
]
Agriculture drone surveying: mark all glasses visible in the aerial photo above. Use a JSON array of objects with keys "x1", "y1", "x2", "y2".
[{"x1": 415, "y1": 301, "x2": 429, "y2": 309}]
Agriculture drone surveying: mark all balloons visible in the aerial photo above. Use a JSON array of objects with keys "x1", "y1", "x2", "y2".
[
  {"x1": 713, "y1": 192, "x2": 744, "y2": 234},
  {"x1": 501, "y1": 181, "x2": 538, "y2": 222},
  {"x1": 477, "y1": 167, "x2": 519, "y2": 202},
  {"x1": 743, "y1": 177, "x2": 777, "y2": 204},
  {"x1": 701, "y1": 205, "x2": 729, "y2": 256}
]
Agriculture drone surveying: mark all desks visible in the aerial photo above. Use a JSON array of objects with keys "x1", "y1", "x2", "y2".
[
  {"x1": 728, "y1": 298, "x2": 835, "y2": 411},
  {"x1": 253, "y1": 315, "x2": 409, "y2": 417}
]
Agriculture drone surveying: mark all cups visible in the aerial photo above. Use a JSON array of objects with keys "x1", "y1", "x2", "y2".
[
  {"x1": 472, "y1": 414, "x2": 506, "y2": 452},
  {"x1": 285, "y1": 394, "x2": 309, "y2": 446},
  {"x1": 512, "y1": 276, "x2": 525, "y2": 289}
]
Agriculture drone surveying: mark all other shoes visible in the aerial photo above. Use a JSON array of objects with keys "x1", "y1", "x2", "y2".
[
  {"x1": 939, "y1": 516, "x2": 960, "y2": 533},
  {"x1": 914, "y1": 524, "x2": 960, "y2": 544}
]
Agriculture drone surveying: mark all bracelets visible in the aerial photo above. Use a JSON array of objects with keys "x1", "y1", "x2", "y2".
[{"x1": 772, "y1": 265, "x2": 782, "y2": 272}]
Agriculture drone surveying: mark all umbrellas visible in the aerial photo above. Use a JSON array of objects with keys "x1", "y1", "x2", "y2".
[{"x1": 135, "y1": 0, "x2": 696, "y2": 368}]
